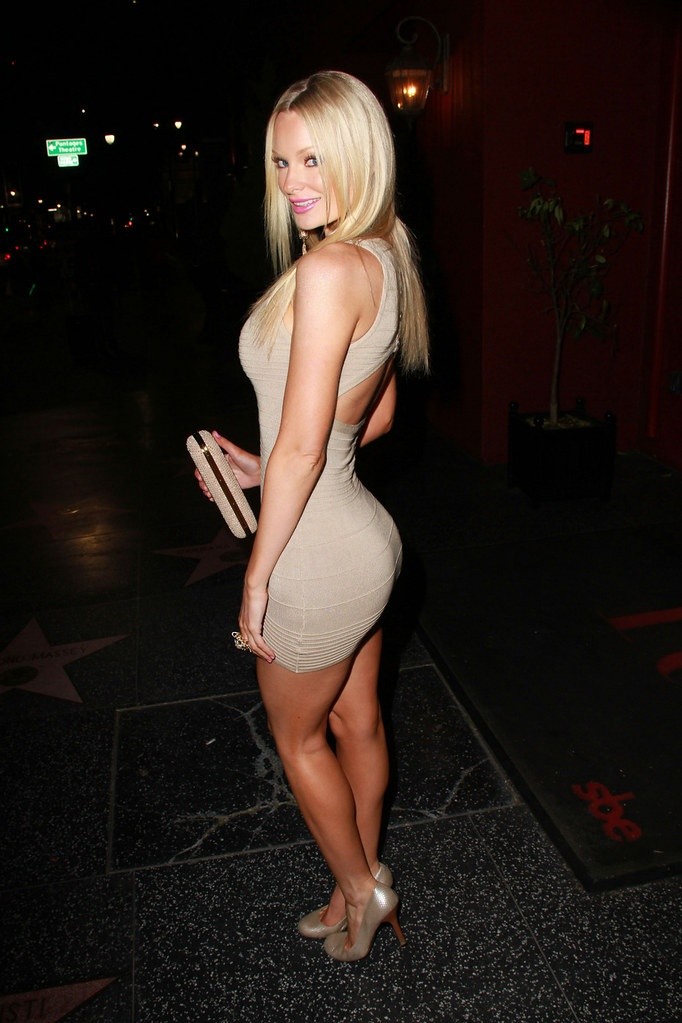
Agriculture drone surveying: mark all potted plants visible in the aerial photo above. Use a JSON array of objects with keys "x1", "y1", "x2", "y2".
[{"x1": 508, "y1": 165, "x2": 645, "y2": 509}]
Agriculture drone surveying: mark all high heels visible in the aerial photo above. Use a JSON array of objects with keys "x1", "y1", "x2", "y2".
[
  {"x1": 299, "y1": 863, "x2": 393, "y2": 938},
  {"x1": 324, "y1": 882, "x2": 405, "y2": 961}
]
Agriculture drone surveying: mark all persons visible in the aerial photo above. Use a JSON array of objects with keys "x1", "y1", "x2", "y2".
[{"x1": 238, "y1": 70, "x2": 430, "y2": 963}]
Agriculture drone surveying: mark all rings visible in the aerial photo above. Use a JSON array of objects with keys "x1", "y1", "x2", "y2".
[{"x1": 232, "y1": 632, "x2": 249, "y2": 651}]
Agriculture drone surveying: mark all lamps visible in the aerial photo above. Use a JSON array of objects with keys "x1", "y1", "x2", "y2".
[{"x1": 376, "y1": 17, "x2": 449, "y2": 132}]
